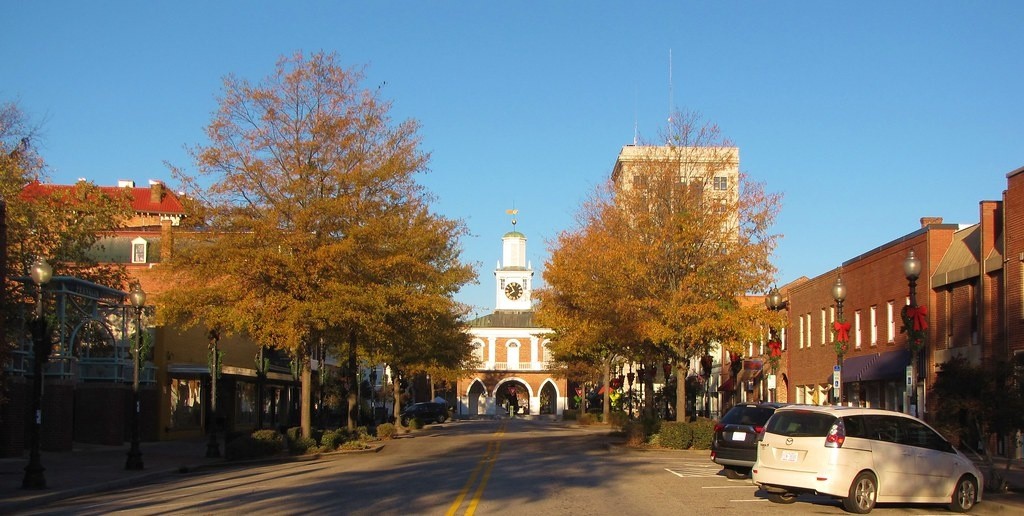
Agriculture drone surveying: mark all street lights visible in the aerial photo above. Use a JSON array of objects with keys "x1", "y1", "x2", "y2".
[
  {"x1": 904, "y1": 247, "x2": 918, "y2": 418},
  {"x1": 769, "y1": 283, "x2": 782, "y2": 402},
  {"x1": 638, "y1": 350, "x2": 643, "y2": 410},
  {"x1": 618, "y1": 361, "x2": 624, "y2": 411},
  {"x1": 19, "y1": 254, "x2": 53, "y2": 492},
  {"x1": 123, "y1": 278, "x2": 147, "y2": 473},
  {"x1": 832, "y1": 275, "x2": 848, "y2": 407},
  {"x1": 627, "y1": 358, "x2": 634, "y2": 416}
]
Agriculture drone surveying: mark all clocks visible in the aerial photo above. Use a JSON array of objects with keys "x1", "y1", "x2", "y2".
[{"x1": 505, "y1": 283, "x2": 522, "y2": 300}]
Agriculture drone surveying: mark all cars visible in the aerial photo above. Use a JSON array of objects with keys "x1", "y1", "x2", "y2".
[
  {"x1": 750, "y1": 403, "x2": 984, "y2": 514},
  {"x1": 388, "y1": 402, "x2": 449, "y2": 427},
  {"x1": 709, "y1": 402, "x2": 793, "y2": 476}
]
